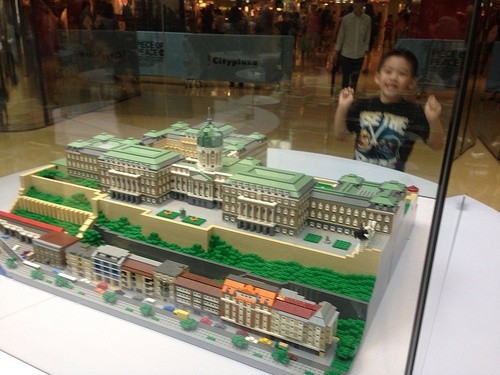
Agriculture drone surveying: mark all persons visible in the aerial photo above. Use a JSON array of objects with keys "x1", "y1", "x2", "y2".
[
  {"x1": 79, "y1": 1, "x2": 403, "y2": 91},
  {"x1": 333, "y1": 48, "x2": 442, "y2": 173}
]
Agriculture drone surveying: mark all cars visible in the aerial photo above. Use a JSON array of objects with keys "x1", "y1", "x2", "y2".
[
  {"x1": 200, "y1": 317, "x2": 297, "y2": 361},
  {"x1": 1, "y1": 235, "x2": 35, "y2": 260},
  {"x1": 95, "y1": 282, "x2": 175, "y2": 313}
]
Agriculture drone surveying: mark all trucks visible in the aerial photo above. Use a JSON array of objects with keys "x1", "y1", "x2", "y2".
[{"x1": 173, "y1": 310, "x2": 189, "y2": 319}]
